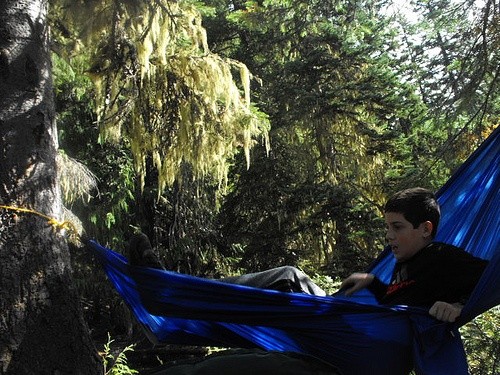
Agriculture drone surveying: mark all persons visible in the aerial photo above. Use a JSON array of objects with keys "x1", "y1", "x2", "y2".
[{"x1": 127, "y1": 184, "x2": 500, "y2": 375}]
